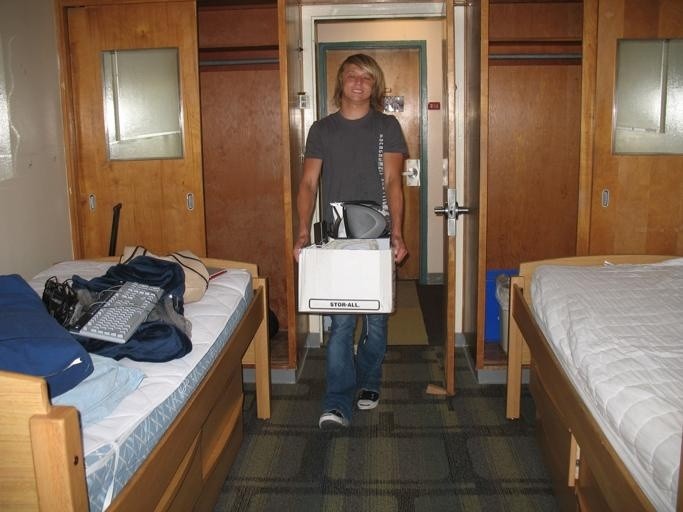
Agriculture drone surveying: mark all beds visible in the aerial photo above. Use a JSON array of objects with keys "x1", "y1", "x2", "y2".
[
  {"x1": 503, "y1": 252, "x2": 682, "y2": 512},
  {"x1": 0, "y1": 255, "x2": 271, "y2": 512}
]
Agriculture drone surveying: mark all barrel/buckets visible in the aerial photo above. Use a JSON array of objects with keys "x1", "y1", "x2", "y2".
[{"x1": 485, "y1": 268, "x2": 521, "y2": 344}]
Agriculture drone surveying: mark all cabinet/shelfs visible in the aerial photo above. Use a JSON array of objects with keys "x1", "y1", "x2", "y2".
[
  {"x1": 463, "y1": 1, "x2": 683, "y2": 371},
  {"x1": 56, "y1": 0, "x2": 302, "y2": 369}
]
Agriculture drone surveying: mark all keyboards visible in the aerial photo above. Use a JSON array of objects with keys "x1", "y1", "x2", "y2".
[{"x1": 69, "y1": 280, "x2": 164, "y2": 345}]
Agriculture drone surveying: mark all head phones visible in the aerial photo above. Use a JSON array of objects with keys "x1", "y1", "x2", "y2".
[{"x1": 42, "y1": 275, "x2": 84, "y2": 326}]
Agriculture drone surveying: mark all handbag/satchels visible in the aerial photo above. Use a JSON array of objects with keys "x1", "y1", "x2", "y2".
[{"x1": 122, "y1": 246, "x2": 210, "y2": 304}]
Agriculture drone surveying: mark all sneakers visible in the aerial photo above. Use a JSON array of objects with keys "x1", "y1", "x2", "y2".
[
  {"x1": 357, "y1": 390, "x2": 379, "y2": 410},
  {"x1": 319, "y1": 410, "x2": 349, "y2": 431}
]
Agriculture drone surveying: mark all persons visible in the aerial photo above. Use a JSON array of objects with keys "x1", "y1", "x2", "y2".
[{"x1": 291, "y1": 53, "x2": 411, "y2": 431}]
